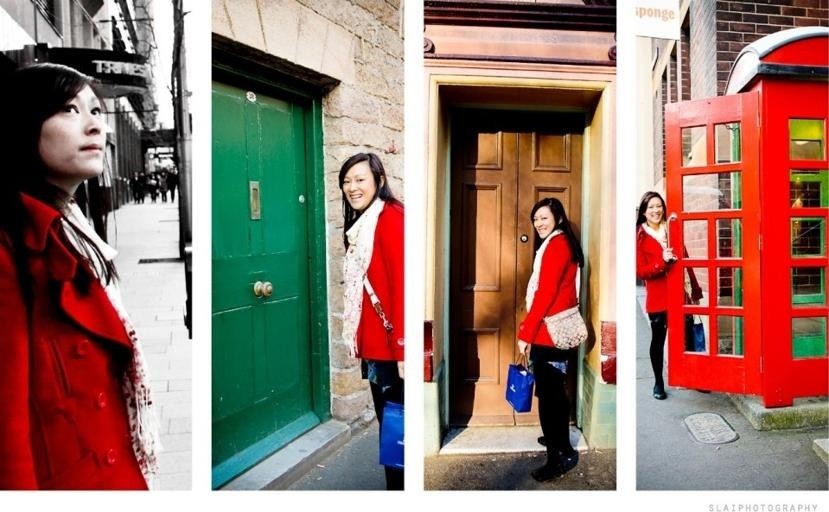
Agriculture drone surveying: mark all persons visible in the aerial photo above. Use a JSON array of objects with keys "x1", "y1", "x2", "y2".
[
  {"x1": 126, "y1": 168, "x2": 180, "y2": 204},
  {"x1": 0, "y1": 63, "x2": 151, "y2": 490},
  {"x1": 519, "y1": 195, "x2": 592, "y2": 482},
  {"x1": 338, "y1": 153, "x2": 405, "y2": 489},
  {"x1": 636, "y1": 190, "x2": 714, "y2": 397}
]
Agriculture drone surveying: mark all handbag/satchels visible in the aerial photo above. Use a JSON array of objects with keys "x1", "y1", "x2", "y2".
[
  {"x1": 503, "y1": 348, "x2": 537, "y2": 415},
  {"x1": 690, "y1": 315, "x2": 706, "y2": 353},
  {"x1": 375, "y1": 397, "x2": 409, "y2": 470}
]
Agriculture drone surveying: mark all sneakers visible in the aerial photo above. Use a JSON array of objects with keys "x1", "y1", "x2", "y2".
[
  {"x1": 529, "y1": 433, "x2": 578, "y2": 481},
  {"x1": 652, "y1": 378, "x2": 670, "y2": 403}
]
